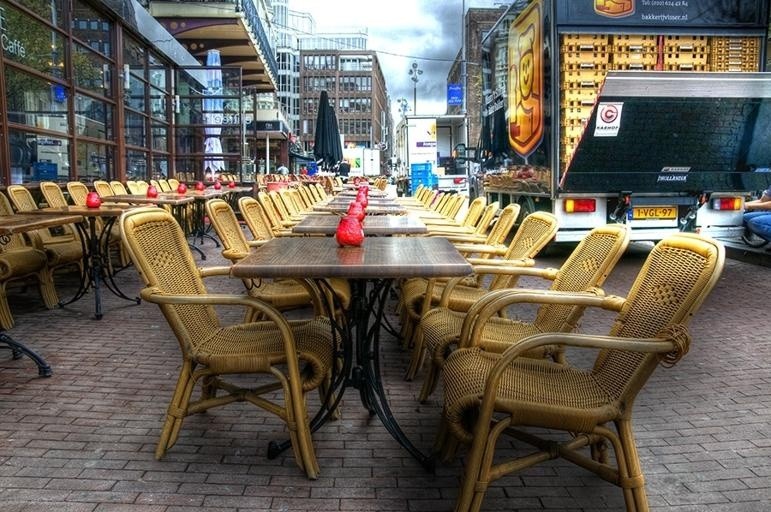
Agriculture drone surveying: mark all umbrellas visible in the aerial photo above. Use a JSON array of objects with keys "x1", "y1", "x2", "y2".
[{"x1": 313, "y1": 90, "x2": 343, "y2": 170}]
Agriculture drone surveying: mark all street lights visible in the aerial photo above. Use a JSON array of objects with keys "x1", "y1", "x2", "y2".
[
  {"x1": 409, "y1": 60, "x2": 423, "y2": 119},
  {"x1": 397, "y1": 96, "x2": 408, "y2": 125}
]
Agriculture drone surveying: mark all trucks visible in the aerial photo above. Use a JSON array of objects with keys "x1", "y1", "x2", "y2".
[
  {"x1": 453, "y1": 0, "x2": 770, "y2": 256},
  {"x1": 391, "y1": 113, "x2": 470, "y2": 198}
]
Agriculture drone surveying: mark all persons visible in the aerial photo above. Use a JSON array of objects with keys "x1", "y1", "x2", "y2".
[{"x1": 743, "y1": 185, "x2": 771, "y2": 252}]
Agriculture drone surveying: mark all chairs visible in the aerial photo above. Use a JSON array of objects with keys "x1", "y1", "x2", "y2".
[
  {"x1": 389, "y1": 183, "x2": 727, "y2": 496},
  {"x1": 1, "y1": 178, "x2": 199, "y2": 327},
  {"x1": 118, "y1": 208, "x2": 339, "y2": 479},
  {"x1": 203, "y1": 182, "x2": 352, "y2": 325}
]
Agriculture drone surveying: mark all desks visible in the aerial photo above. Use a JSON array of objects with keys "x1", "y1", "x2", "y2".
[
  {"x1": 292, "y1": 188, "x2": 427, "y2": 237},
  {"x1": 232, "y1": 236, "x2": 474, "y2": 477}
]
